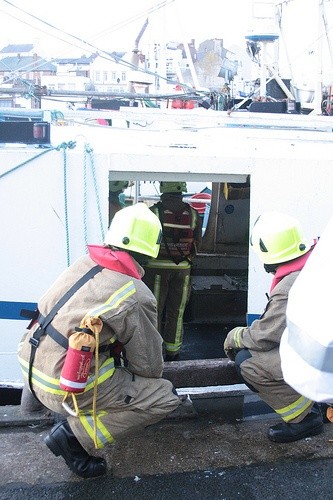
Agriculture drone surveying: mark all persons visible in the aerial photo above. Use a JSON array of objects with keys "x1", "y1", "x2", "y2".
[
  {"x1": 210, "y1": 92, "x2": 230, "y2": 110},
  {"x1": 15, "y1": 202, "x2": 180, "y2": 478},
  {"x1": 138, "y1": 181, "x2": 202, "y2": 361},
  {"x1": 224, "y1": 212, "x2": 333, "y2": 444}
]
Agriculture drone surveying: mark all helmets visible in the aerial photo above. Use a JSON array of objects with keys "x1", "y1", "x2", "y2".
[
  {"x1": 106, "y1": 203, "x2": 162, "y2": 258},
  {"x1": 158, "y1": 181, "x2": 187, "y2": 192},
  {"x1": 254, "y1": 215, "x2": 311, "y2": 265},
  {"x1": 109, "y1": 181, "x2": 127, "y2": 191}
]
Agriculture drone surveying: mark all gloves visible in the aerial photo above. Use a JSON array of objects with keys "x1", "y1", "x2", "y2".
[{"x1": 223, "y1": 326, "x2": 245, "y2": 362}]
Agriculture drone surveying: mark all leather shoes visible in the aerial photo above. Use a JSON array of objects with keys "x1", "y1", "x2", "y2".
[
  {"x1": 268, "y1": 411, "x2": 323, "y2": 441},
  {"x1": 46, "y1": 421, "x2": 107, "y2": 478}
]
